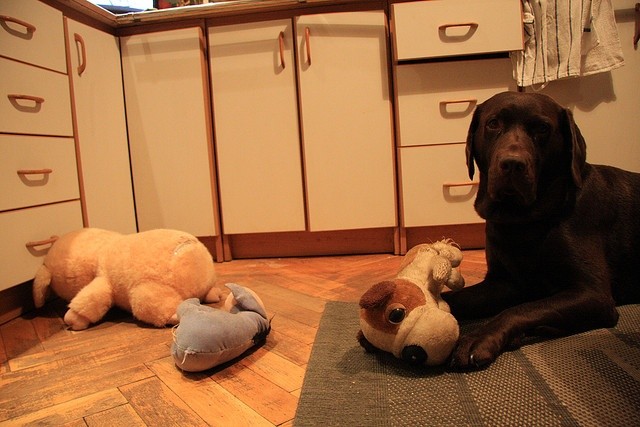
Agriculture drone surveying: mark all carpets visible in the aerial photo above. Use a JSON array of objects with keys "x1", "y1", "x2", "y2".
[{"x1": 293, "y1": 298, "x2": 640, "y2": 427}]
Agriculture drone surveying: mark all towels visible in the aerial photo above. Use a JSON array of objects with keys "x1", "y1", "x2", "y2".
[{"x1": 509, "y1": 0, "x2": 625, "y2": 92}]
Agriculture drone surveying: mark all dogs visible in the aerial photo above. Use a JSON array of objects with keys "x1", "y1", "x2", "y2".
[{"x1": 356, "y1": 91, "x2": 640, "y2": 374}]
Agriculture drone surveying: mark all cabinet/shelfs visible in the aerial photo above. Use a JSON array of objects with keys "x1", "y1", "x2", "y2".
[
  {"x1": 1, "y1": 1, "x2": 86, "y2": 329},
  {"x1": 389, "y1": 0, "x2": 525, "y2": 255},
  {"x1": 119, "y1": 26, "x2": 224, "y2": 263},
  {"x1": 64, "y1": 16, "x2": 139, "y2": 233},
  {"x1": 205, "y1": 1, "x2": 400, "y2": 261}
]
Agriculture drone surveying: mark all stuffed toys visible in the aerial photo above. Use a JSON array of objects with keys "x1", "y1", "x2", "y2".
[
  {"x1": 32, "y1": 227, "x2": 222, "y2": 331},
  {"x1": 171, "y1": 282, "x2": 272, "y2": 373},
  {"x1": 355, "y1": 235, "x2": 466, "y2": 366}
]
